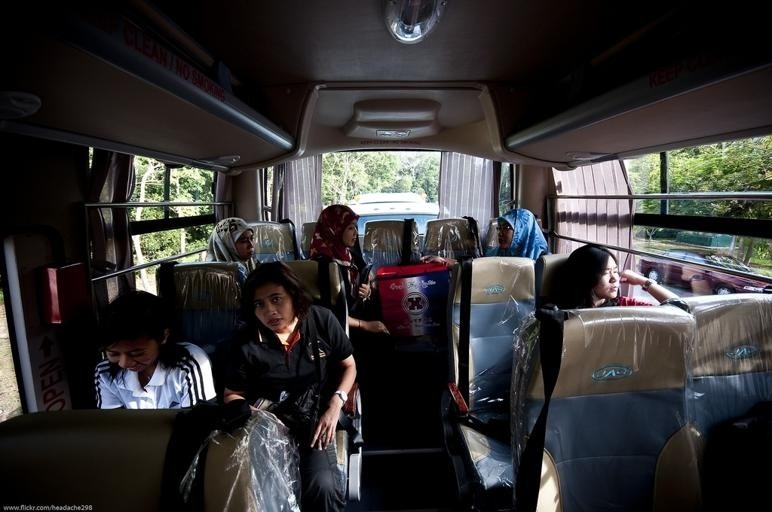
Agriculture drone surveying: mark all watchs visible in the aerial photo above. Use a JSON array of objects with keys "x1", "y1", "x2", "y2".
[{"x1": 334, "y1": 389, "x2": 350, "y2": 404}]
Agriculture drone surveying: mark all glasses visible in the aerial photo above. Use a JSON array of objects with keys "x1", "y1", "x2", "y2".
[{"x1": 496, "y1": 225, "x2": 511, "y2": 231}]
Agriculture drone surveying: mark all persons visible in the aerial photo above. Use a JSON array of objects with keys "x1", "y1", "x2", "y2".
[
  {"x1": 559, "y1": 245, "x2": 680, "y2": 311},
  {"x1": 419, "y1": 208, "x2": 552, "y2": 269},
  {"x1": 205, "y1": 218, "x2": 258, "y2": 287},
  {"x1": 94, "y1": 290, "x2": 218, "y2": 411},
  {"x1": 219, "y1": 260, "x2": 359, "y2": 511},
  {"x1": 308, "y1": 203, "x2": 401, "y2": 405}
]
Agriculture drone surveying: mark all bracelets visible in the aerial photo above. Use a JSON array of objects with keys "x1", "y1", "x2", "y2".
[
  {"x1": 358, "y1": 319, "x2": 363, "y2": 329},
  {"x1": 643, "y1": 277, "x2": 655, "y2": 291}
]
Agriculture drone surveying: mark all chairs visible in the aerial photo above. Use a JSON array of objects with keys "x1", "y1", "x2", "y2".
[{"x1": 0, "y1": 215, "x2": 771, "y2": 511}]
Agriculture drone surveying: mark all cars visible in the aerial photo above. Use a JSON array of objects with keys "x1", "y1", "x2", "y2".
[
  {"x1": 344, "y1": 202, "x2": 449, "y2": 237},
  {"x1": 637, "y1": 247, "x2": 771, "y2": 295}
]
